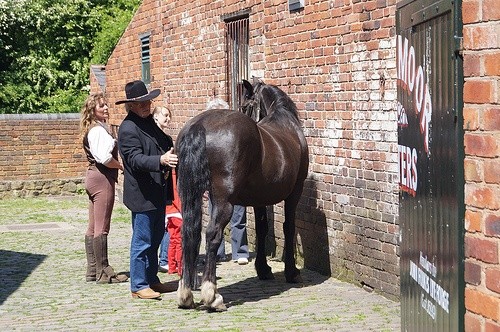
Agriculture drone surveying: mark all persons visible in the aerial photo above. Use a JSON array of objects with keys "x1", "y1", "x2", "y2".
[
  {"x1": 152, "y1": 105, "x2": 184, "y2": 275},
  {"x1": 79, "y1": 93, "x2": 130, "y2": 284},
  {"x1": 207, "y1": 99, "x2": 250, "y2": 265},
  {"x1": 114, "y1": 80, "x2": 178, "y2": 300}
]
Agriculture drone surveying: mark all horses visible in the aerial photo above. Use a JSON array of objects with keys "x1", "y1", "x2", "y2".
[{"x1": 173, "y1": 75, "x2": 310, "y2": 310}]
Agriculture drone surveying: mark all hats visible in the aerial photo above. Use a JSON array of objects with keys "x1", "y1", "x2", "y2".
[{"x1": 115, "y1": 80, "x2": 161, "y2": 105}]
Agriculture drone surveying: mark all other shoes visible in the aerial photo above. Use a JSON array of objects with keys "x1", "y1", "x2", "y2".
[
  {"x1": 158, "y1": 262, "x2": 179, "y2": 273},
  {"x1": 153, "y1": 282, "x2": 177, "y2": 294},
  {"x1": 132, "y1": 288, "x2": 161, "y2": 299},
  {"x1": 238, "y1": 257, "x2": 248, "y2": 264}
]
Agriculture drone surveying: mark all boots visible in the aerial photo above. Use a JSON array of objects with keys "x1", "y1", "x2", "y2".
[
  {"x1": 85, "y1": 235, "x2": 96, "y2": 281},
  {"x1": 92, "y1": 232, "x2": 128, "y2": 284}
]
